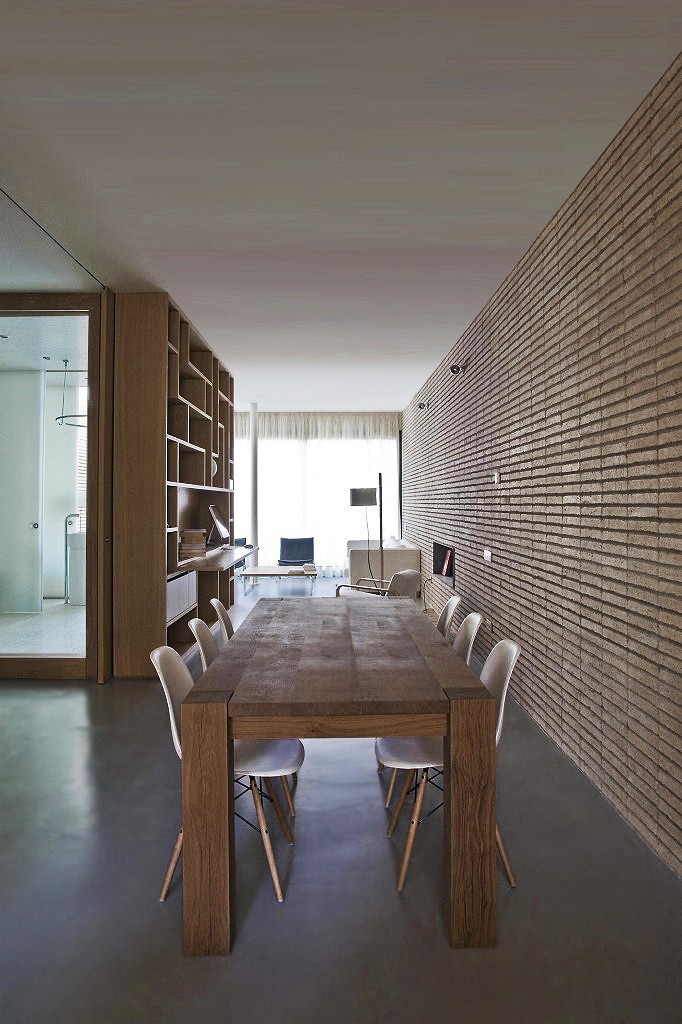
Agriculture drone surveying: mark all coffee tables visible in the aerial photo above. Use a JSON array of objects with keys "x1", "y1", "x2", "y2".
[{"x1": 240, "y1": 566, "x2": 318, "y2": 599}]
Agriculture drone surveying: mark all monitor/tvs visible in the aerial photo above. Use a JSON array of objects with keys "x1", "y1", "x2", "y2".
[{"x1": 205, "y1": 505, "x2": 230, "y2": 545}]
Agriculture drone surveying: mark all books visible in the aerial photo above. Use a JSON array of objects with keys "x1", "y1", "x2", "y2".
[
  {"x1": 178, "y1": 528, "x2": 207, "y2": 557},
  {"x1": 287, "y1": 563, "x2": 316, "y2": 574}
]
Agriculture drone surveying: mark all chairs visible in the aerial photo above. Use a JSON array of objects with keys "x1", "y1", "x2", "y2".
[
  {"x1": 149, "y1": 646, "x2": 296, "y2": 903},
  {"x1": 209, "y1": 597, "x2": 234, "y2": 645},
  {"x1": 188, "y1": 618, "x2": 304, "y2": 818},
  {"x1": 436, "y1": 596, "x2": 461, "y2": 638},
  {"x1": 377, "y1": 611, "x2": 485, "y2": 810},
  {"x1": 374, "y1": 638, "x2": 521, "y2": 892},
  {"x1": 335, "y1": 569, "x2": 420, "y2": 598},
  {"x1": 233, "y1": 537, "x2": 247, "y2": 571},
  {"x1": 277, "y1": 537, "x2": 317, "y2": 567}
]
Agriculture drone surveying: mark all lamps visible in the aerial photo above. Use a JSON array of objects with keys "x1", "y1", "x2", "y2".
[
  {"x1": 418, "y1": 402, "x2": 430, "y2": 409},
  {"x1": 450, "y1": 364, "x2": 466, "y2": 375}
]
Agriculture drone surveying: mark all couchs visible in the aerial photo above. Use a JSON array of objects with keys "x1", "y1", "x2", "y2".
[{"x1": 345, "y1": 536, "x2": 421, "y2": 598}]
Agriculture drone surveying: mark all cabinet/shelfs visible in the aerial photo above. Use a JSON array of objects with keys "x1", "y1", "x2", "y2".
[{"x1": 112, "y1": 291, "x2": 262, "y2": 679}]
